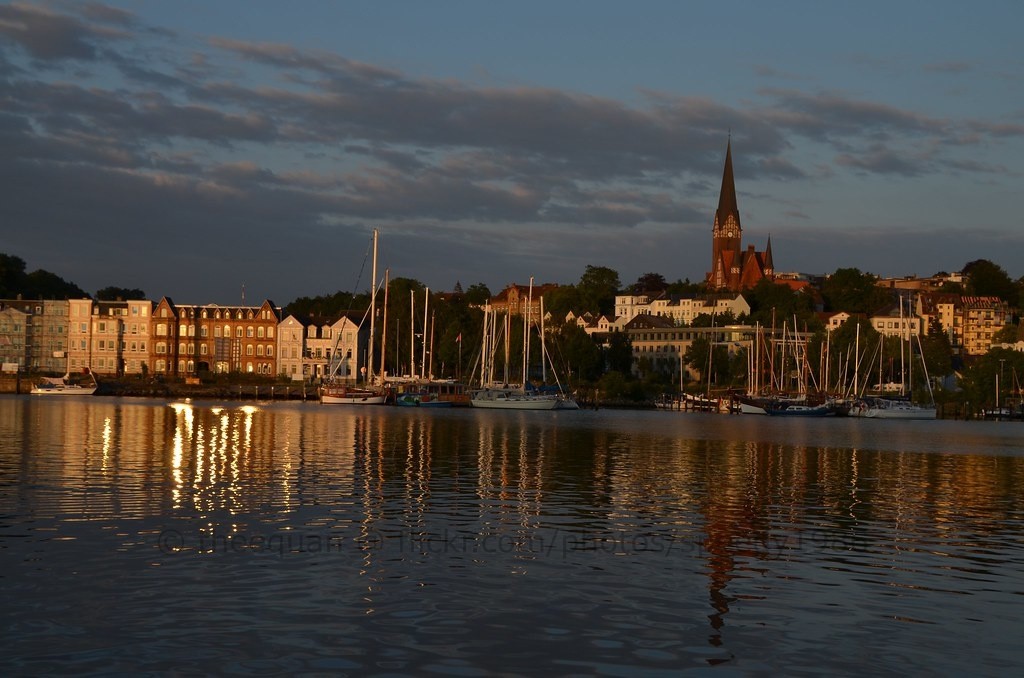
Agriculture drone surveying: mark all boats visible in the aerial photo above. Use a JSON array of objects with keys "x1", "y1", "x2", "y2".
[
  {"x1": 653, "y1": 380, "x2": 1024, "y2": 419},
  {"x1": 317, "y1": 370, "x2": 582, "y2": 411},
  {"x1": 30, "y1": 365, "x2": 98, "y2": 395}
]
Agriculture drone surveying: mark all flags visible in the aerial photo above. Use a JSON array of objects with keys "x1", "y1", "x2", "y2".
[{"x1": 455, "y1": 334, "x2": 461, "y2": 342}]
